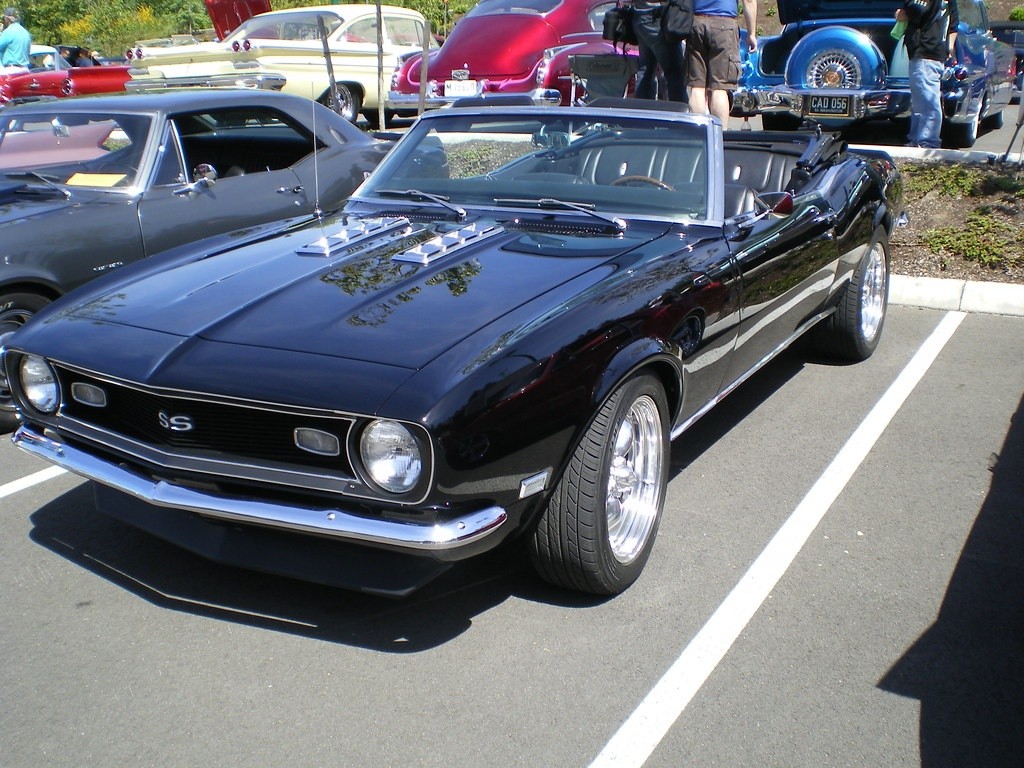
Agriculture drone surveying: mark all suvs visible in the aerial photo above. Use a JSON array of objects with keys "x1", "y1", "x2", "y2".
[{"x1": 125, "y1": 4, "x2": 441, "y2": 126}]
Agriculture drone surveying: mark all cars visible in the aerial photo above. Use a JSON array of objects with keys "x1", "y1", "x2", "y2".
[
  {"x1": 976, "y1": 19, "x2": 1023, "y2": 104},
  {"x1": 0, "y1": 0, "x2": 282, "y2": 110},
  {"x1": 0, "y1": 73, "x2": 449, "y2": 436}
]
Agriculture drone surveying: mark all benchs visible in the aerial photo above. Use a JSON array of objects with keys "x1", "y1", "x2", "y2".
[
  {"x1": 575, "y1": 133, "x2": 800, "y2": 196},
  {"x1": 121, "y1": 133, "x2": 308, "y2": 176}
]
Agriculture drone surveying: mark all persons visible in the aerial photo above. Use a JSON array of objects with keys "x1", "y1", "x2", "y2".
[
  {"x1": 895, "y1": 0, "x2": 959, "y2": 149},
  {"x1": 75, "y1": 46, "x2": 94, "y2": 67},
  {"x1": 631, "y1": 0, "x2": 689, "y2": 105},
  {"x1": 685, "y1": 0, "x2": 758, "y2": 132},
  {"x1": 42, "y1": 47, "x2": 71, "y2": 68},
  {"x1": 0, "y1": 7, "x2": 31, "y2": 75}
]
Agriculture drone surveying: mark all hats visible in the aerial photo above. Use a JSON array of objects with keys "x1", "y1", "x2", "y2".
[{"x1": 4, "y1": 7, "x2": 18, "y2": 16}]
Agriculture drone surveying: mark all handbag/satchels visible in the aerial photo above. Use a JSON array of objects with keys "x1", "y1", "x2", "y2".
[
  {"x1": 660, "y1": 0, "x2": 694, "y2": 40},
  {"x1": 890, "y1": 18, "x2": 908, "y2": 40},
  {"x1": 603, "y1": 0, "x2": 638, "y2": 46}
]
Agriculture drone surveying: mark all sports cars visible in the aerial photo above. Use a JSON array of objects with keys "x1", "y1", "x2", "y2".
[
  {"x1": 377, "y1": 0, "x2": 668, "y2": 135},
  {"x1": 727, "y1": 0, "x2": 1018, "y2": 148},
  {"x1": 0, "y1": 92, "x2": 911, "y2": 600}
]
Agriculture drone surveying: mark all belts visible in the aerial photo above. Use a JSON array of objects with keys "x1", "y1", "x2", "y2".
[{"x1": 695, "y1": 13, "x2": 735, "y2": 19}]
